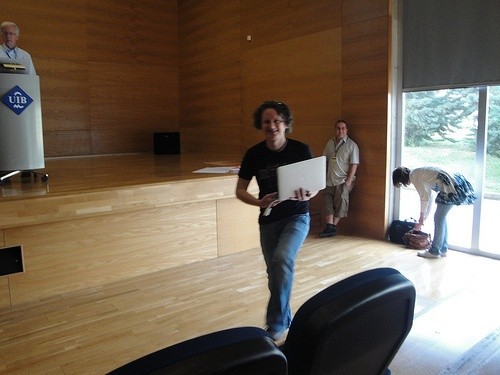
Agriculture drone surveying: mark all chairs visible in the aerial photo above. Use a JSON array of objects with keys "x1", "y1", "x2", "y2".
[
  {"x1": 284, "y1": 266, "x2": 417, "y2": 375},
  {"x1": 103, "y1": 323, "x2": 290, "y2": 375}
]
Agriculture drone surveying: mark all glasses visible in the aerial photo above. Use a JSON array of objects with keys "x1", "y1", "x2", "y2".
[{"x1": 263, "y1": 120, "x2": 284, "y2": 125}]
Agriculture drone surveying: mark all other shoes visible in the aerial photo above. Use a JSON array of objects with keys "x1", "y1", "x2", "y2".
[
  {"x1": 417, "y1": 251, "x2": 441, "y2": 259},
  {"x1": 264, "y1": 327, "x2": 289, "y2": 347},
  {"x1": 428, "y1": 248, "x2": 449, "y2": 257},
  {"x1": 318, "y1": 227, "x2": 336, "y2": 237}
]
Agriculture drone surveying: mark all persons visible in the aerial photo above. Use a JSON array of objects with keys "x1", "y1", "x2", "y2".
[
  {"x1": 320, "y1": 120, "x2": 359, "y2": 237},
  {"x1": 0, "y1": 22, "x2": 37, "y2": 75},
  {"x1": 392, "y1": 166, "x2": 478, "y2": 258},
  {"x1": 236, "y1": 102, "x2": 319, "y2": 339}
]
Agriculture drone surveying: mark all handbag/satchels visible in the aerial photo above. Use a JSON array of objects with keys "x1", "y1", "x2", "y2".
[
  {"x1": 390, "y1": 220, "x2": 416, "y2": 244},
  {"x1": 402, "y1": 230, "x2": 431, "y2": 248}
]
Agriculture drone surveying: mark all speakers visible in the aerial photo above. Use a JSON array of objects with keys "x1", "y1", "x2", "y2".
[{"x1": 153, "y1": 132, "x2": 180, "y2": 155}]
